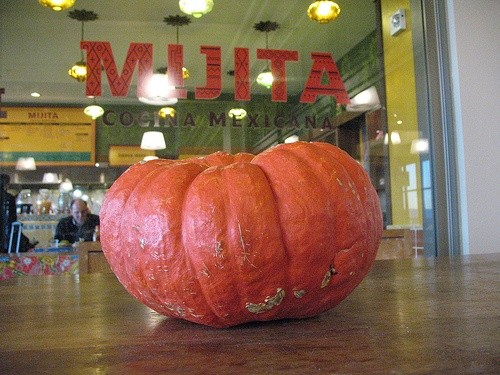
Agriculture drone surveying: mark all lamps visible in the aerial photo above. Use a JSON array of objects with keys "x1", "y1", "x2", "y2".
[
  {"x1": 84, "y1": 97, "x2": 105, "y2": 120},
  {"x1": 39, "y1": 0, "x2": 77, "y2": 12},
  {"x1": 252, "y1": 19, "x2": 281, "y2": 90},
  {"x1": 140, "y1": 131, "x2": 167, "y2": 162},
  {"x1": 179, "y1": 0, "x2": 215, "y2": 19},
  {"x1": 162, "y1": 14, "x2": 193, "y2": 79},
  {"x1": 67, "y1": 8, "x2": 99, "y2": 83},
  {"x1": 306, "y1": 0, "x2": 341, "y2": 24}
]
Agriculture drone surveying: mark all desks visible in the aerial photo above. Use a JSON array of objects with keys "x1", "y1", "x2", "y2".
[{"x1": 0, "y1": 251, "x2": 500, "y2": 375}]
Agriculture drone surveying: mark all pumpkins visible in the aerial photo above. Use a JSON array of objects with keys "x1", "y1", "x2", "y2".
[{"x1": 99, "y1": 141, "x2": 382, "y2": 327}]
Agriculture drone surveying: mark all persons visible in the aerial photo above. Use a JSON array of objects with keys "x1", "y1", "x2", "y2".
[
  {"x1": 54, "y1": 199, "x2": 100, "y2": 244},
  {"x1": 0, "y1": 174, "x2": 17, "y2": 253}
]
